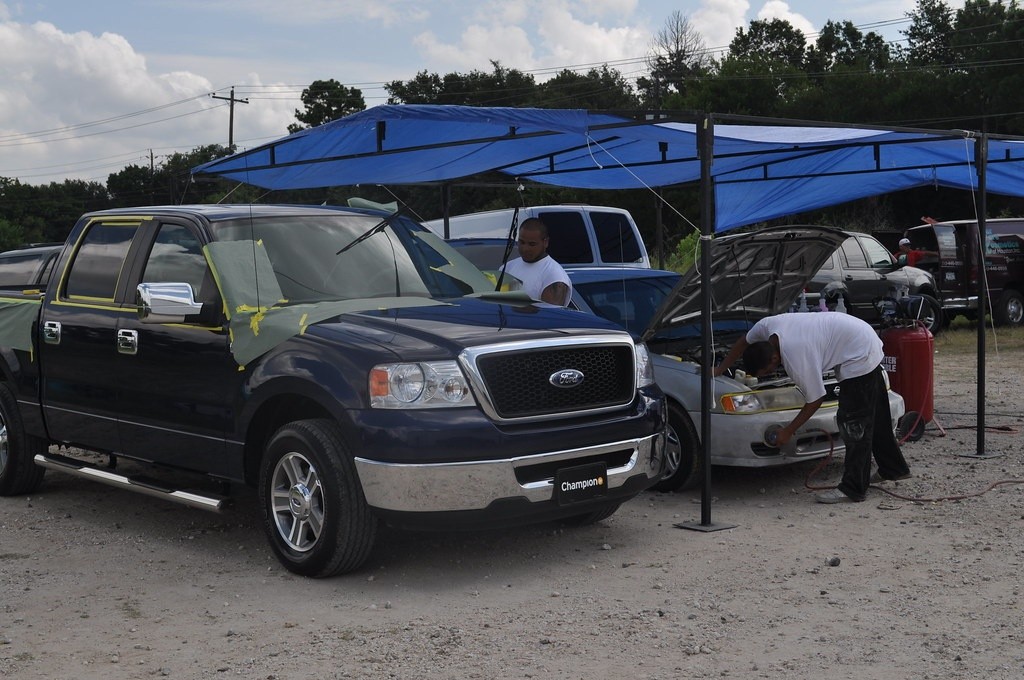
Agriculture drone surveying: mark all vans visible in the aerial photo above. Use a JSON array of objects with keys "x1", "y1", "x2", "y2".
[
  {"x1": 419, "y1": 203, "x2": 650, "y2": 320},
  {"x1": 872, "y1": 218, "x2": 1024, "y2": 328}
]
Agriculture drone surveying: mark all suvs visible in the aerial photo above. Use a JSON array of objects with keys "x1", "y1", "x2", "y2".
[{"x1": 788, "y1": 227, "x2": 944, "y2": 335}]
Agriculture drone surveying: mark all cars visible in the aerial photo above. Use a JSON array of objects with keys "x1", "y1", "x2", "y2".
[{"x1": 564, "y1": 224, "x2": 905, "y2": 493}]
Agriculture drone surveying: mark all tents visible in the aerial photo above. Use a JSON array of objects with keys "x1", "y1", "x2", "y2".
[{"x1": 190, "y1": 103, "x2": 1023, "y2": 533}]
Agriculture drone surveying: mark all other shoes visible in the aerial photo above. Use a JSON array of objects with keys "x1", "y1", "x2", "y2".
[
  {"x1": 869, "y1": 466, "x2": 911, "y2": 483},
  {"x1": 816, "y1": 485, "x2": 865, "y2": 503}
]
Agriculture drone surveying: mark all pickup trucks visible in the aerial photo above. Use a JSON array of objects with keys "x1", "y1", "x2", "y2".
[{"x1": 0, "y1": 204, "x2": 668, "y2": 579}]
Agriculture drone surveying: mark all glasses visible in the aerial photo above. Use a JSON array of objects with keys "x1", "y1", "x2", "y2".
[{"x1": 903, "y1": 244, "x2": 910, "y2": 246}]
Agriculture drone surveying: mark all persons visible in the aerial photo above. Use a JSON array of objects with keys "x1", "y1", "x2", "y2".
[
  {"x1": 498, "y1": 218, "x2": 572, "y2": 307},
  {"x1": 695, "y1": 312, "x2": 912, "y2": 503},
  {"x1": 893, "y1": 238, "x2": 940, "y2": 269}
]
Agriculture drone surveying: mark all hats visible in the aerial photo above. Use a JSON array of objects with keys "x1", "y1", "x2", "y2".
[{"x1": 899, "y1": 238, "x2": 911, "y2": 246}]
